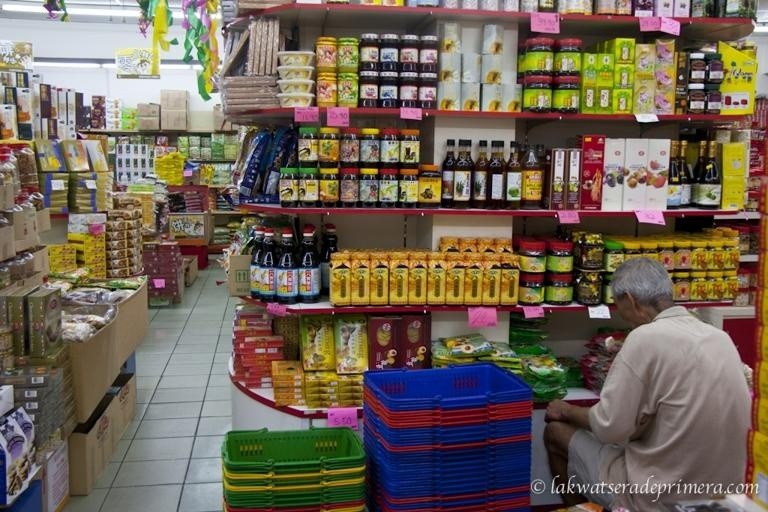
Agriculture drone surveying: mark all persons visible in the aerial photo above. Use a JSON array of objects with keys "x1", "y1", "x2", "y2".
[{"x1": 543, "y1": 256, "x2": 752, "y2": 512}]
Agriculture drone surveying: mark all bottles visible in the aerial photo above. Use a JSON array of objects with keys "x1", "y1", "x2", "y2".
[
  {"x1": 437, "y1": 138, "x2": 551, "y2": 206},
  {"x1": 248, "y1": 225, "x2": 339, "y2": 303},
  {"x1": 662, "y1": 138, "x2": 722, "y2": 209}
]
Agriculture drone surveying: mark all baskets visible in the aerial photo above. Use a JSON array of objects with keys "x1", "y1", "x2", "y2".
[
  {"x1": 221, "y1": 427, "x2": 365, "y2": 512},
  {"x1": 363, "y1": 364, "x2": 533, "y2": 512}
]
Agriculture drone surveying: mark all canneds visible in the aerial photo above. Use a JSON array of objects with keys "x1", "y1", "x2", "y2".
[
  {"x1": 315, "y1": 33, "x2": 439, "y2": 109},
  {"x1": 512, "y1": 223, "x2": 759, "y2": 308},
  {"x1": 518, "y1": 37, "x2": 583, "y2": 115},
  {"x1": 280, "y1": 126, "x2": 441, "y2": 208}
]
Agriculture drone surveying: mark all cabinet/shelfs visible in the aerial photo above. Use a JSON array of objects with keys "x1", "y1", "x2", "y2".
[
  {"x1": 712, "y1": 207, "x2": 757, "y2": 361},
  {"x1": 217, "y1": 0, "x2": 751, "y2": 505}
]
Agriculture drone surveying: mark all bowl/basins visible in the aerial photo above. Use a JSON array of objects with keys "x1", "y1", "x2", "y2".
[{"x1": 277, "y1": 50, "x2": 316, "y2": 107}]
